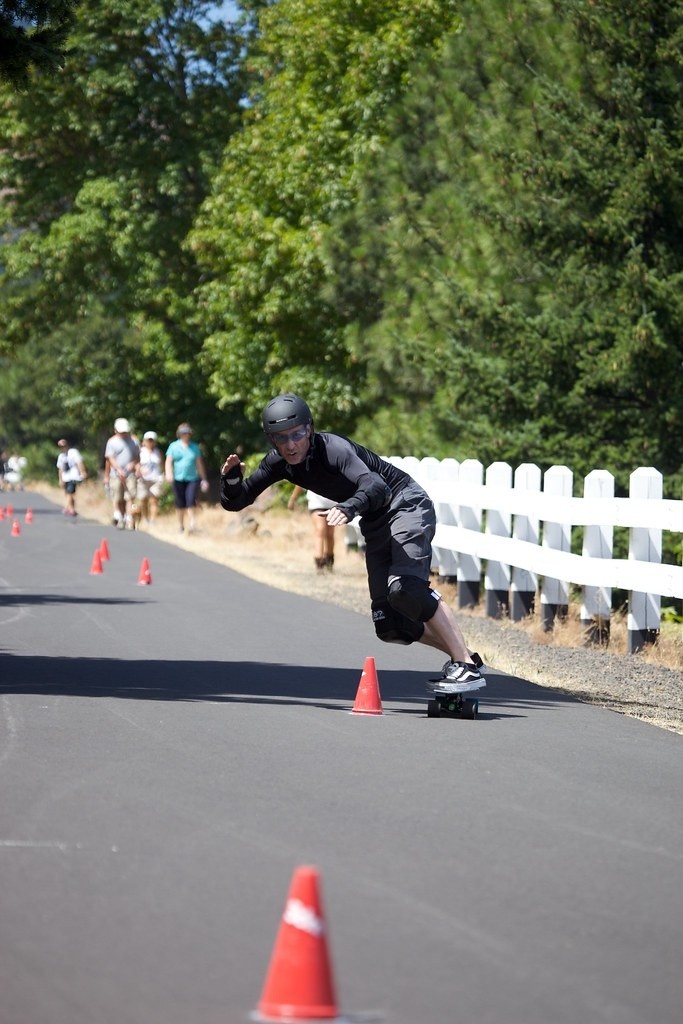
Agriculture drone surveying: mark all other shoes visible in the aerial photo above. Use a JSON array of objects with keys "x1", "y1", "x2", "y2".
[
  {"x1": 64, "y1": 508, "x2": 69, "y2": 515},
  {"x1": 69, "y1": 510, "x2": 77, "y2": 516}
]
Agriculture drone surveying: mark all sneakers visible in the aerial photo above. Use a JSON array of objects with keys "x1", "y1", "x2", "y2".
[
  {"x1": 426, "y1": 652, "x2": 486, "y2": 686},
  {"x1": 113, "y1": 518, "x2": 135, "y2": 531},
  {"x1": 439, "y1": 661, "x2": 486, "y2": 686}
]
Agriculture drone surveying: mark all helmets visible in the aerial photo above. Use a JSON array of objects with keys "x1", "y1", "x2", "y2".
[{"x1": 262, "y1": 395, "x2": 314, "y2": 449}]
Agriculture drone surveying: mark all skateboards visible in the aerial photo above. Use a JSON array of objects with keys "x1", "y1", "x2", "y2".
[{"x1": 422, "y1": 686, "x2": 480, "y2": 720}]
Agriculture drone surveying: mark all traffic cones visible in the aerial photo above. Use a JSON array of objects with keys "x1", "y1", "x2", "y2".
[
  {"x1": 25, "y1": 505, "x2": 33, "y2": 524},
  {"x1": 254, "y1": 865, "x2": 342, "y2": 1022},
  {"x1": 7, "y1": 503, "x2": 14, "y2": 516},
  {"x1": 137, "y1": 559, "x2": 152, "y2": 586},
  {"x1": 0, "y1": 504, "x2": 7, "y2": 521},
  {"x1": 352, "y1": 657, "x2": 384, "y2": 714},
  {"x1": 92, "y1": 540, "x2": 110, "y2": 575},
  {"x1": 11, "y1": 518, "x2": 24, "y2": 536}
]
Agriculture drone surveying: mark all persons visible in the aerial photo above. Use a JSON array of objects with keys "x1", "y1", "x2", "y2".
[
  {"x1": 56, "y1": 439, "x2": 87, "y2": 517},
  {"x1": 103, "y1": 418, "x2": 208, "y2": 538},
  {"x1": 287, "y1": 484, "x2": 338, "y2": 574},
  {"x1": 0, "y1": 450, "x2": 28, "y2": 492},
  {"x1": 220, "y1": 394, "x2": 486, "y2": 693}
]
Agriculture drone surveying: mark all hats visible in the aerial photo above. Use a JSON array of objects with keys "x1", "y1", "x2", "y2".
[
  {"x1": 143, "y1": 431, "x2": 157, "y2": 442},
  {"x1": 176, "y1": 423, "x2": 192, "y2": 435},
  {"x1": 114, "y1": 418, "x2": 131, "y2": 433}
]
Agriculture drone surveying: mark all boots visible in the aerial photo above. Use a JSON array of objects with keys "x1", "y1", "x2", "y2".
[
  {"x1": 316, "y1": 558, "x2": 325, "y2": 577},
  {"x1": 324, "y1": 555, "x2": 334, "y2": 576}
]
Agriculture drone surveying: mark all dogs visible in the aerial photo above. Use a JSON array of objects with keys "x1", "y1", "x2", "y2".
[{"x1": 127, "y1": 505, "x2": 142, "y2": 531}]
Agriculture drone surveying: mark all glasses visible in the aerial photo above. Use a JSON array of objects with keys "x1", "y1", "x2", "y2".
[{"x1": 274, "y1": 424, "x2": 307, "y2": 444}]
